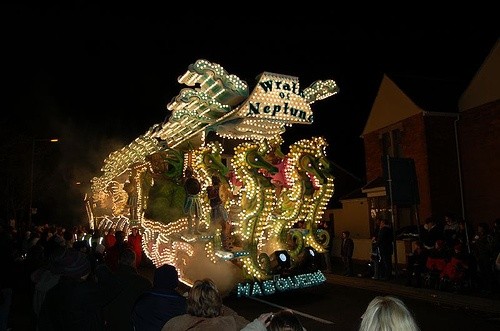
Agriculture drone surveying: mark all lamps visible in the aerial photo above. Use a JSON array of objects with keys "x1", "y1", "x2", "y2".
[
  {"x1": 295, "y1": 246, "x2": 320, "y2": 274},
  {"x1": 268, "y1": 250, "x2": 291, "y2": 274}
]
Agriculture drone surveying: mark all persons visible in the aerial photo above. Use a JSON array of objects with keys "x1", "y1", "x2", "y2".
[
  {"x1": 161, "y1": 278, "x2": 249, "y2": 331},
  {"x1": 358, "y1": 296, "x2": 420, "y2": 331},
  {"x1": 341, "y1": 231, "x2": 354, "y2": 275},
  {"x1": 296, "y1": 221, "x2": 329, "y2": 273},
  {"x1": 409, "y1": 213, "x2": 500, "y2": 300},
  {"x1": 0, "y1": 211, "x2": 142, "y2": 307},
  {"x1": 65, "y1": 260, "x2": 137, "y2": 331},
  {"x1": 368, "y1": 210, "x2": 394, "y2": 280},
  {"x1": 132, "y1": 264, "x2": 187, "y2": 331},
  {"x1": 240, "y1": 309, "x2": 307, "y2": 331},
  {"x1": 9, "y1": 244, "x2": 89, "y2": 331}
]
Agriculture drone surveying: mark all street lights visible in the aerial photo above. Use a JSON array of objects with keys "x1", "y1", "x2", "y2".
[{"x1": 28, "y1": 137, "x2": 60, "y2": 223}]
